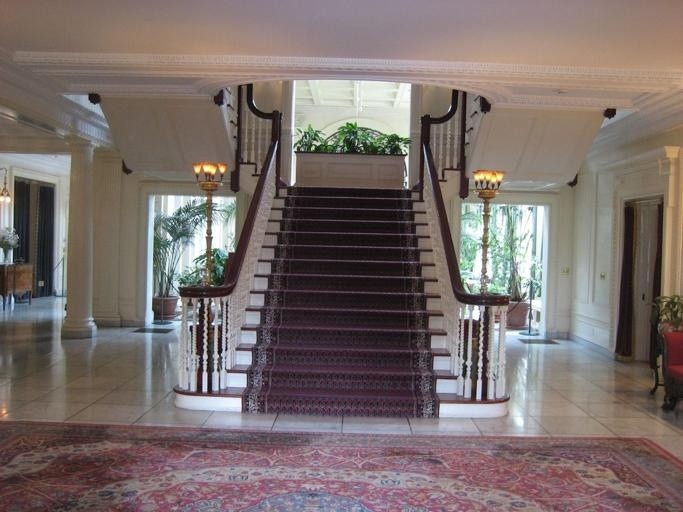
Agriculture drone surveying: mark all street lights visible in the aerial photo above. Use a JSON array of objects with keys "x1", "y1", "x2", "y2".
[
  {"x1": 472, "y1": 168, "x2": 505, "y2": 294},
  {"x1": 191, "y1": 160, "x2": 228, "y2": 289}
]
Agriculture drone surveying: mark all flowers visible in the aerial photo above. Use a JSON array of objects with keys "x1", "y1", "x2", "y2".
[{"x1": 0, "y1": 225, "x2": 19, "y2": 263}]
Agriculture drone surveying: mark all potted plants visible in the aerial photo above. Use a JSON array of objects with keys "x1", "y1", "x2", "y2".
[
  {"x1": 293, "y1": 122, "x2": 412, "y2": 189},
  {"x1": 496, "y1": 206, "x2": 532, "y2": 331},
  {"x1": 645, "y1": 289, "x2": 682, "y2": 337},
  {"x1": 153, "y1": 202, "x2": 216, "y2": 320}
]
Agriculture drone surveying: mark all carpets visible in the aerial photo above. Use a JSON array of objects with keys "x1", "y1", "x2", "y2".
[{"x1": 0, "y1": 419, "x2": 682, "y2": 511}]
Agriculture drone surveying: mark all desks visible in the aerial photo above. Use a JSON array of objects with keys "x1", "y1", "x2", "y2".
[{"x1": 0, "y1": 263, "x2": 36, "y2": 310}]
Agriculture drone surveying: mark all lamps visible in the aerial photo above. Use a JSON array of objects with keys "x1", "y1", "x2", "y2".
[
  {"x1": 471, "y1": 167, "x2": 505, "y2": 400},
  {"x1": 190, "y1": 161, "x2": 227, "y2": 391}
]
